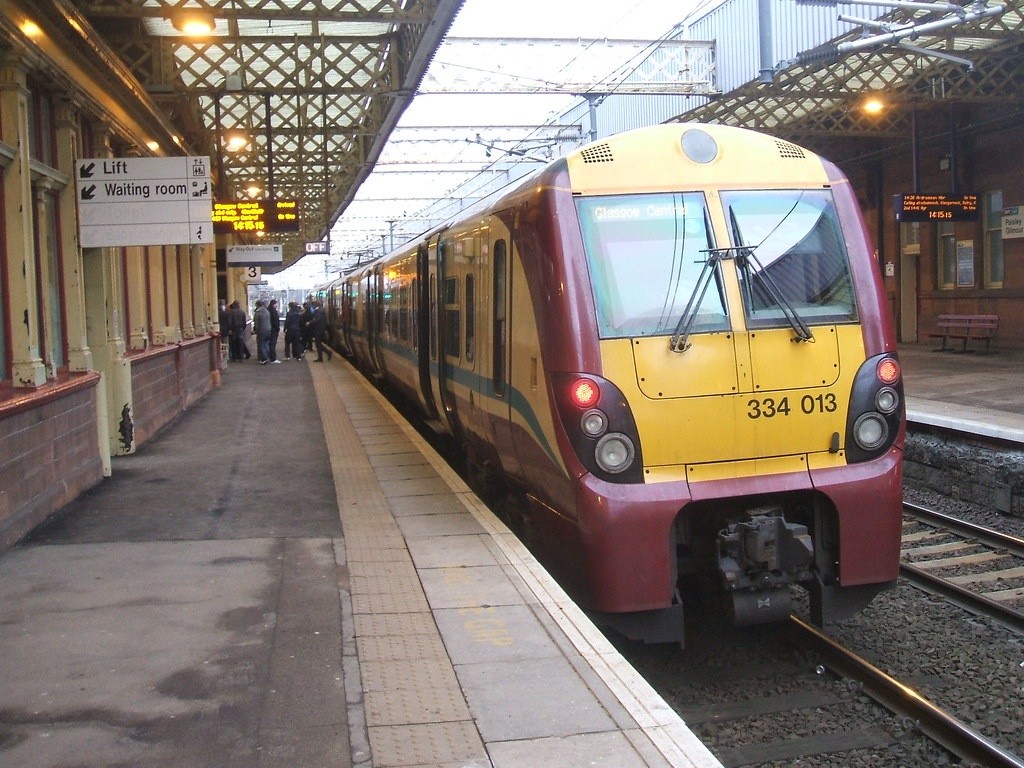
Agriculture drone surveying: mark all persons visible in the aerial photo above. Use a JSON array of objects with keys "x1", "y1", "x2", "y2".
[
  {"x1": 218, "y1": 299, "x2": 229, "y2": 339},
  {"x1": 282, "y1": 300, "x2": 332, "y2": 362},
  {"x1": 251, "y1": 300, "x2": 272, "y2": 364},
  {"x1": 228, "y1": 300, "x2": 251, "y2": 362},
  {"x1": 268, "y1": 299, "x2": 282, "y2": 364}
]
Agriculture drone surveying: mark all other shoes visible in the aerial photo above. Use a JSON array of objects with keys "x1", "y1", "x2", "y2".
[
  {"x1": 270, "y1": 360, "x2": 282, "y2": 364},
  {"x1": 306, "y1": 345, "x2": 313, "y2": 352},
  {"x1": 244, "y1": 353, "x2": 251, "y2": 360},
  {"x1": 260, "y1": 359, "x2": 268, "y2": 365},
  {"x1": 314, "y1": 358, "x2": 324, "y2": 362},
  {"x1": 328, "y1": 351, "x2": 332, "y2": 361},
  {"x1": 300, "y1": 349, "x2": 309, "y2": 358},
  {"x1": 283, "y1": 357, "x2": 290, "y2": 361},
  {"x1": 229, "y1": 358, "x2": 236, "y2": 363},
  {"x1": 298, "y1": 358, "x2": 301, "y2": 361},
  {"x1": 238, "y1": 358, "x2": 242, "y2": 363}
]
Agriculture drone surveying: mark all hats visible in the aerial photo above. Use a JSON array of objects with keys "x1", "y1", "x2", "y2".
[{"x1": 229, "y1": 300, "x2": 240, "y2": 309}]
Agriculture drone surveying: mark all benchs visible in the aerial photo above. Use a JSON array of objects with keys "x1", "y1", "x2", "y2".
[{"x1": 927, "y1": 313, "x2": 1001, "y2": 356}]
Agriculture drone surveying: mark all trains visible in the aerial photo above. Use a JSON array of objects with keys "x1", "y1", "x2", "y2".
[{"x1": 315, "y1": 123, "x2": 908, "y2": 658}]
